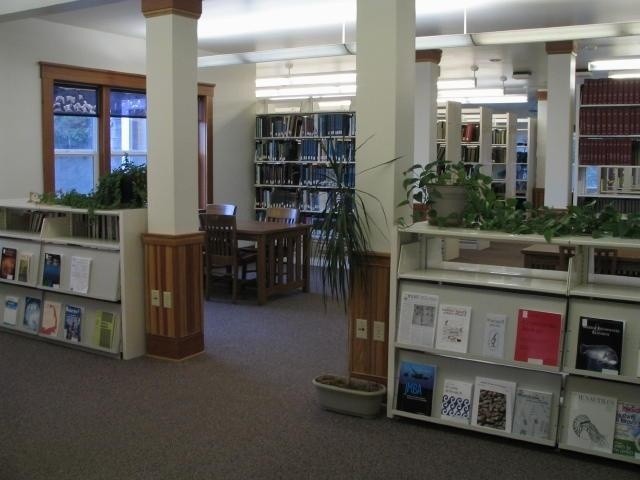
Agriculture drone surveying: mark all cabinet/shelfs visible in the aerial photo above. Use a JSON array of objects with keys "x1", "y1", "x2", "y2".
[
  {"x1": 574, "y1": 104, "x2": 640, "y2": 221},
  {"x1": 255, "y1": 114, "x2": 359, "y2": 268},
  {"x1": 0, "y1": 198, "x2": 147, "y2": 361},
  {"x1": 385, "y1": 221, "x2": 639, "y2": 467},
  {"x1": 430, "y1": 119, "x2": 527, "y2": 203}
]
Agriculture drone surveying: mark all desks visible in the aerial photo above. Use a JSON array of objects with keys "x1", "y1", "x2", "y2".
[{"x1": 233, "y1": 219, "x2": 315, "y2": 303}]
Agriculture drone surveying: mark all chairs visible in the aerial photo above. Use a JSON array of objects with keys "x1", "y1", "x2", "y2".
[
  {"x1": 262, "y1": 206, "x2": 301, "y2": 292},
  {"x1": 201, "y1": 205, "x2": 236, "y2": 218},
  {"x1": 198, "y1": 213, "x2": 257, "y2": 301}
]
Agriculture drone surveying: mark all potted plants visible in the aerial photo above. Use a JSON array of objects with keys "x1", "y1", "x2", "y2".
[
  {"x1": 282, "y1": 116, "x2": 385, "y2": 417},
  {"x1": 31, "y1": 151, "x2": 146, "y2": 226},
  {"x1": 393, "y1": 159, "x2": 640, "y2": 239}
]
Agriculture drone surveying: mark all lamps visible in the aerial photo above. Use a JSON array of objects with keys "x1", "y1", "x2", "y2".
[
  {"x1": 255, "y1": 74, "x2": 529, "y2": 104},
  {"x1": 586, "y1": 54, "x2": 639, "y2": 79}
]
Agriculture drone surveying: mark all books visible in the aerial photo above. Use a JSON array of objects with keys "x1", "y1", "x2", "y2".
[
  {"x1": 0, "y1": 206, "x2": 120, "y2": 355},
  {"x1": 439, "y1": 376, "x2": 552, "y2": 441},
  {"x1": 566, "y1": 391, "x2": 640, "y2": 460},
  {"x1": 254, "y1": 112, "x2": 356, "y2": 239},
  {"x1": 437, "y1": 123, "x2": 507, "y2": 201},
  {"x1": 398, "y1": 291, "x2": 472, "y2": 353},
  {"x1": 578, "y1": 77, "x2": 640, "y2": 167},
  {"x1": 575, "y1": 316, "x2": 626, "y2": 376},
  {"x1": 396, "y1": 361, "x2": 439, "y2": 418},
  {"x1": 514, "y1": 309, "x2": 562, "y2": 366}
]
